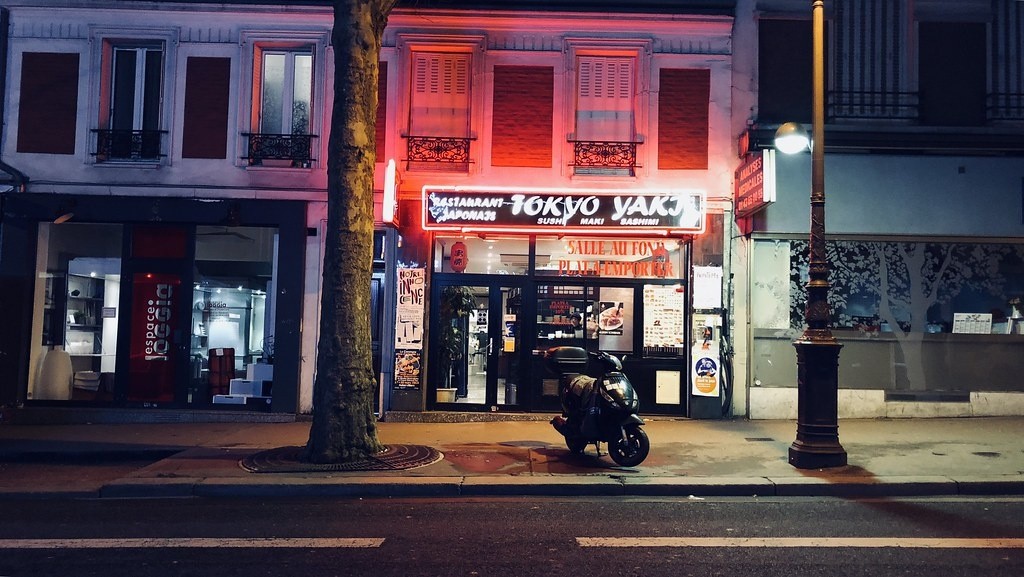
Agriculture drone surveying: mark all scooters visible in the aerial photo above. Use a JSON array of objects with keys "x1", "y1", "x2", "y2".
[{"x1": 542, "y1": 346, "x2": 653, "y2": 468}]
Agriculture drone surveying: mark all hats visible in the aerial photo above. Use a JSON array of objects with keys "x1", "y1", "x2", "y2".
[{"x1": 570, "y1": 313, "x2": 581, "y2": 321}]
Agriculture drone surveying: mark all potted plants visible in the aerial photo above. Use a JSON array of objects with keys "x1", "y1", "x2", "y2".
[{"x1": 436, "y1": 286, "x2": 478, "y2": 403}]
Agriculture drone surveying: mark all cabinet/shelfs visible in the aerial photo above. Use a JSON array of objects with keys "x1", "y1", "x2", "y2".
[
  {"x1": 64, "y1": 272, "x2": 106, "y2": 373},
  {"x1": 41, "y1": 273, "x2": 67, "y2": 347},
  {"x1": 506, "y1": 300, "x2": 594, "y2": 338}
]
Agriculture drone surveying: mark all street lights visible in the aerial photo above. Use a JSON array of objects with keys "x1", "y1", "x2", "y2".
[{"x1": 774, "y1": 123, "x2": 851, "y2": 468}]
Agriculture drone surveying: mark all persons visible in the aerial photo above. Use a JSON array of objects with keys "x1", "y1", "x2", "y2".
[
  {"x1": 569, "y1": 313, "x2": 582, "y2": 335},
  {"x1": 477, "y1": 331, "x2": 488, "y2": 349}
]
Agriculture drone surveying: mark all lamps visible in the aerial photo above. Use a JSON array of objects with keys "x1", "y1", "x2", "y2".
[{"x1": 774, "y1": 122, "x2": 809, "y2": 155}]
